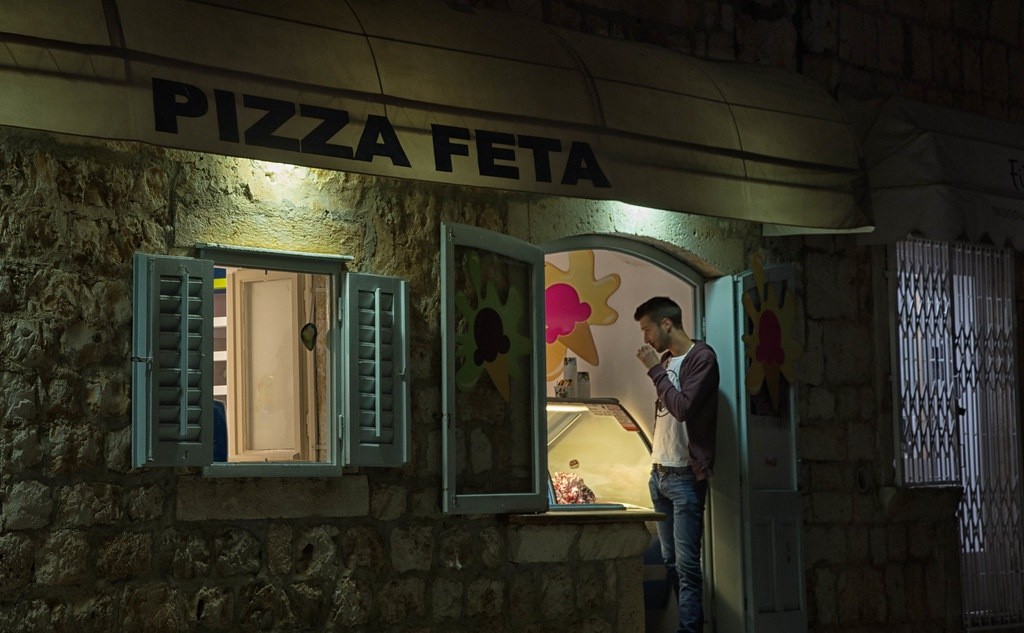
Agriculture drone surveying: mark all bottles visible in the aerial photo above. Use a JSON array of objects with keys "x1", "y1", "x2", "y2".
[
  {"x1": 563, "y1": 357, "x2": 578, "y2": 397},
  {"x1": 578, "y1": 372, "x2": 591, "y2": 398}
]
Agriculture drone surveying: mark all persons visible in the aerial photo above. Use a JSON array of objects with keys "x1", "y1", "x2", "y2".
[{"x1": 632, "y1": 296, "x2": 722, "y2": 632}]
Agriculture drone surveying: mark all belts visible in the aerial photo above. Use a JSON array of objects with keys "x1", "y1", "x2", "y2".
[{"x1": 653, "y1": 464, "x2": 694, "y2": 476}]
